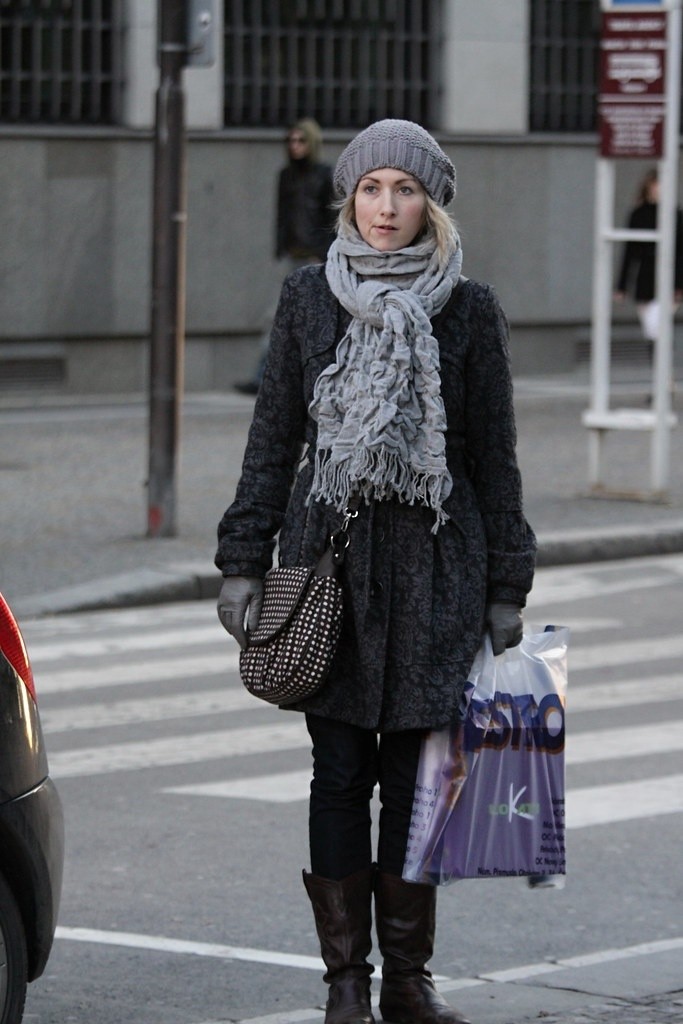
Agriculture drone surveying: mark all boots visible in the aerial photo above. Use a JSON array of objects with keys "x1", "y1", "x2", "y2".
[
  {"x1": 302, "y1": 863, "x2": 375, "y2": 1024},
  {"x1": 373, "y1": 862, "x2": 471, "y2": 1024}
]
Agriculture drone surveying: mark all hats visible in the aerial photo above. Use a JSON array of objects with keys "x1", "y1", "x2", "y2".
[{"x1": 332, "y1": 119, "x2": 456, "y2": 208}]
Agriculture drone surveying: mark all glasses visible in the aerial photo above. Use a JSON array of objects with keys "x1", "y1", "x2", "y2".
[{"x1": 284, "y1": 137, "x2": 306, "y2": 144}]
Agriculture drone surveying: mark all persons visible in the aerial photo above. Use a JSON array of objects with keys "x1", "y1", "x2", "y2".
[
  {"x1": 211, "y1": 116, "x2": 540, "y2": 1024},
  {"x1": 613, "y1": 167, "x2": 683, "y2": 409}
]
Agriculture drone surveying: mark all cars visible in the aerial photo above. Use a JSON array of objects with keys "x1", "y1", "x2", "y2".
[{"x1": 1, "y1": 592, "x2": 65, "y2": 1023}]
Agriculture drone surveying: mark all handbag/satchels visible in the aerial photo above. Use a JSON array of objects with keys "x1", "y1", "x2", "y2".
[
  {"x1": 239, "y1": 567, "x2": 344, "y2": 705},
  {"x1": 402, "y1": 625, "x2": 568, "y2": 891}
]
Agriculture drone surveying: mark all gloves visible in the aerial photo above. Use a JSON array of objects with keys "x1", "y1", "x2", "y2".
[
  {"x1": 217, "y1": 575, "x2": 264, "y2": 650},
  {"x1": 483, "y1": 603, "x2": 523, "y2": 656}
]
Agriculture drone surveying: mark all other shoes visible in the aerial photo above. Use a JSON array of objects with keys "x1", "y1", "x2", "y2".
[{"x1": 234, "y1": 381, "x2": 259, "y2": 394}]
